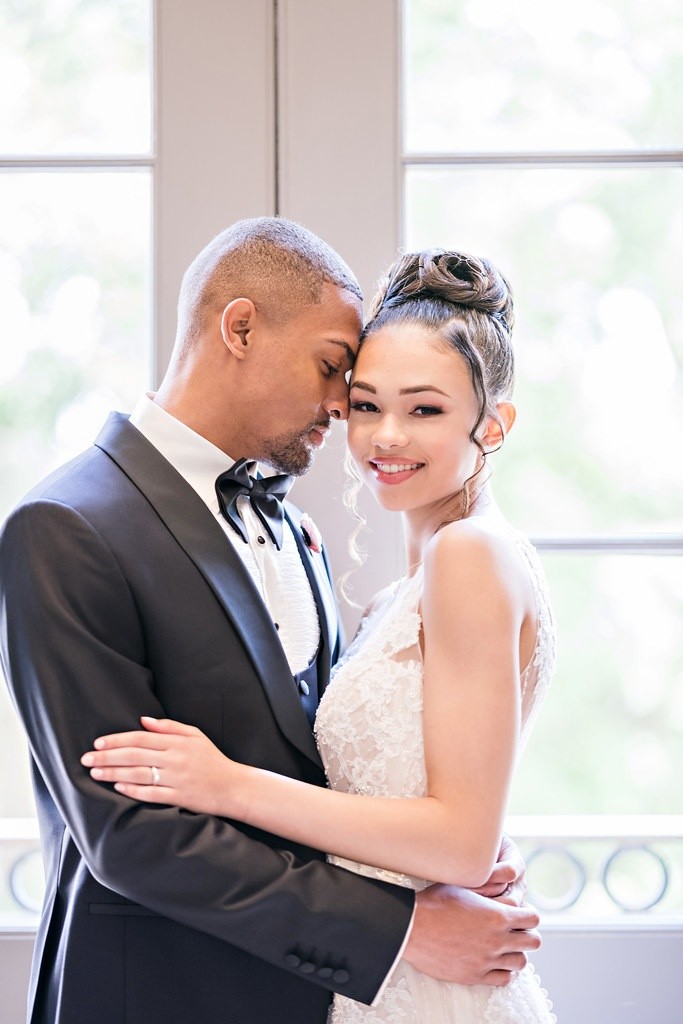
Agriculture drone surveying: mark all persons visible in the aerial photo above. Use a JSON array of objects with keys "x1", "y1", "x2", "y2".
[
  {"x1": 81, "y1": 247, "x2": 562, "y2": 1024},
  {"x1": 0, "y1": 216, "x2": 542, "y2": 1024}
]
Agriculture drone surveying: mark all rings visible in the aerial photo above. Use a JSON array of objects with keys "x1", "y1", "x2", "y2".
[{"x1": 151, "y1": 767, "x2": 159, "y2": 785}]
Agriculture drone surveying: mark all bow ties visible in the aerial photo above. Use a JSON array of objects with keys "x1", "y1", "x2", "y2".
[{"x1": 214, "y1": 456, "x2": 296, "y2": 551}]
